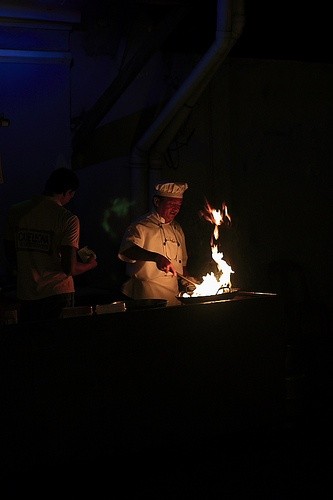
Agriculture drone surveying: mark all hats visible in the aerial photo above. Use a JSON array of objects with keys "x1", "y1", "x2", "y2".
[{"x1": 154, "y1": 182, "x2": 188, "y2": 199}]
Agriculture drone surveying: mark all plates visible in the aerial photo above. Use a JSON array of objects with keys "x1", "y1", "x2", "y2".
[{"x1": 125, "y1": 299, "x2": 167, "y2": 309}]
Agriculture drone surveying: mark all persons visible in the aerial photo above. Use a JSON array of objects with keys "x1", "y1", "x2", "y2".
[
  {"x1": 3, "y1": 165, "x2": 98, "y2": 312},
  {"x1": 117, "y1": 182, "x2": 190, "y2": 301}
]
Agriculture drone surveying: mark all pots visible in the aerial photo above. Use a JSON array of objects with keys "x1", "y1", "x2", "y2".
[{"x1": 175, "y1": 288, "x2": 240, "y2": 303}]
